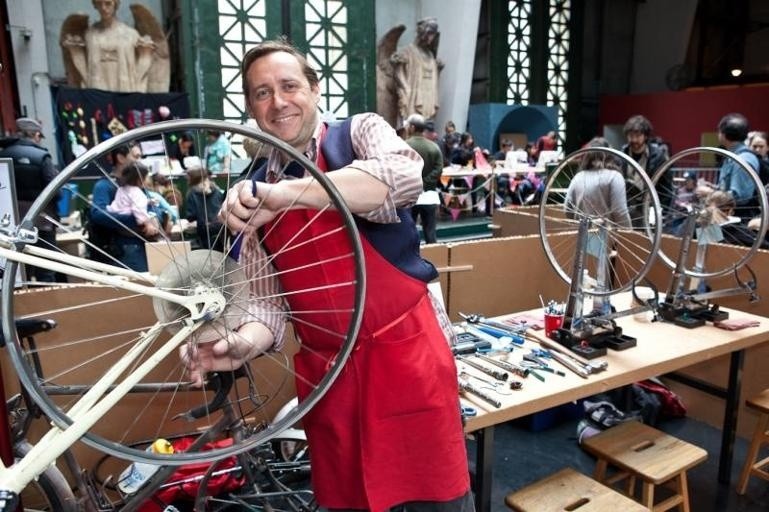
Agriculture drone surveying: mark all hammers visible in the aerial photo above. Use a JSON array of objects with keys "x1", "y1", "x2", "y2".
[{"x1": 525, "y1": 327, "x2": 608, "y2": 373}]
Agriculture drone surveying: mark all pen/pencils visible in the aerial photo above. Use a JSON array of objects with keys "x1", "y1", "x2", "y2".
[
  {"x1": 539, "y1": 295, "x2": 564, "y2": 315},
  {"x1": 477, "y1": 348, "x2": 513, "y2": 352}
]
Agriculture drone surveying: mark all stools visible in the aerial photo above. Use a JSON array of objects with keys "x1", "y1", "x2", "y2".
[
  {"x1": 579, "y1": 418, "x2": 711, "y2": 511},
  {"x1": 735, "y1": 387, "x2": 768, "y2": 495},
  {"x1": 502, "y1": 466, "x2": 653, "y2": 511}
]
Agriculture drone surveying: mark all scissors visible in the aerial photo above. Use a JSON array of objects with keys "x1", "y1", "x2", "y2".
[{"x1": 460, "y1": 403, "x2": 477, "y2": 417}]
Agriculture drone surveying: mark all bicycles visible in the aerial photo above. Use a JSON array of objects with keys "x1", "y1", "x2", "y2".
[
  {"x1": 0, "y1": 312, "x2": 320, "y2": 511},
  {"x1": 0, "y1": 117, "x2": 367, "y2": 512}
]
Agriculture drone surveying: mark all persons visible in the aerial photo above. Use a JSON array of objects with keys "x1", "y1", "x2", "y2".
[
  {"x1": 562, "y1": 113, "x2": 769, "y2": 251},
  {"x1": 179, "y1": 37, "x2": 476, "y2": 512},
  {"x1": 396, "y1": 112, "x2": 557, "y2": 243},
  {"x1": 83, "y1": 0, "x2": 155, "y2": 93},
  {"x1": 80, "y1": 120, "x2": 268, "y2": 259},
  {"x1": 0, "y1": 117, "x2": 64, "y2": 282},
  {"x1": 391, "y1": 19, "x2": 441, "y2": 121}
]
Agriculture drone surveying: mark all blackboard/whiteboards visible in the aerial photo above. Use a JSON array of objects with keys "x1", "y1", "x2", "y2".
[{"x1": 0, "y1": 158, "x2": 28, "y2": 289}]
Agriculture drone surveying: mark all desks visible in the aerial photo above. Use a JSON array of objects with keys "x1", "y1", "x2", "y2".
[
  {"x1": 56, "y1": 155, "x2": 555, "y2": 265},
  {"x1": 449, "y1": 285, "x2": 768, "y2": 512}
]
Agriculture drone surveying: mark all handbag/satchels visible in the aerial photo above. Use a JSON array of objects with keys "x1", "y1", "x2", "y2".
[{"x1": 577, "y1": 401, "x2": 642, "y2": 450}]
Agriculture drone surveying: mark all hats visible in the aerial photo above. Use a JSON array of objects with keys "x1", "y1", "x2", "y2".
[
  {"x1": 187, "y1": 168, "x2": 210, "y2": 185},
  {"x1": 14, "y1": 118, "x2": 45, "y2": 140}
]
